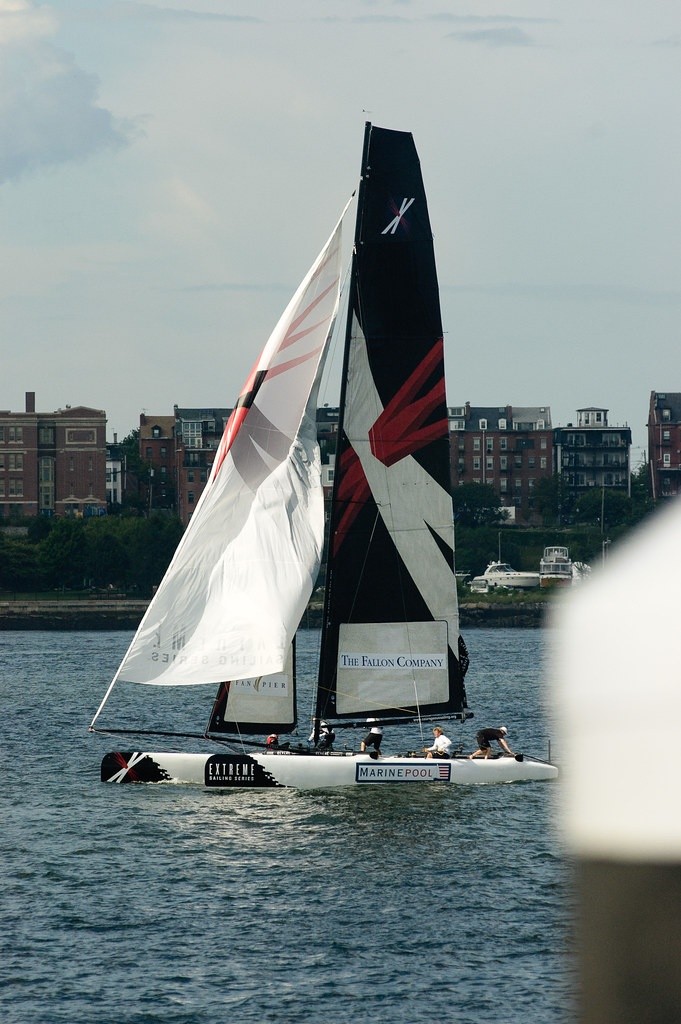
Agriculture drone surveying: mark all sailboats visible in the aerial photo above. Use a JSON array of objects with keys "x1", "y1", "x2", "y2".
[{"x1": 89, "y1": 119, "x2": 561, "y2": 795}]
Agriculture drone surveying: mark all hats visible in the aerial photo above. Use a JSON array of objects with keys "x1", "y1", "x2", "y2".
[
  {"x1": 269, "y1": 734, "x2": 277, "y2": 739},
  {"x1": 500, "y1": 726, "x2": 508, "y2": 735}
]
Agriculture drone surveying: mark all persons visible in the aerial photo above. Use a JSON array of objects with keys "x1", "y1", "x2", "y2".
[
  {"x1": 307, "y1": 717, "x2": 335, "y2": 752},
  {"x1": 420, "y1": 726, "x2": 454, "y2": 760},
  {"x1": 359, "y1": 718, "x2": 385, "y2": 756},
  {"x1": 467, "y1": 726, "x2": 516, "y2": 760},
  {"x1": 265, "y1": 731, "x2": 290, "y2": 752}
]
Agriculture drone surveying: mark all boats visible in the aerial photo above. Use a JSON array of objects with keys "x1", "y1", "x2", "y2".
[
  {"x1": 473, "y1": 533, "x2": 541, "y2": 592},
  {"x1": 455, "y1": 568, "x2": 470, "y2": 581},
  {"x1": 540, "y1": 547, "x2": 573, "y2": 588}
]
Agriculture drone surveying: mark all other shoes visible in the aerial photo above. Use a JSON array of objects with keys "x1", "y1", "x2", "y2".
[{"x1": 468, "y1": 754, "x2": 473, "y2": 759}]
